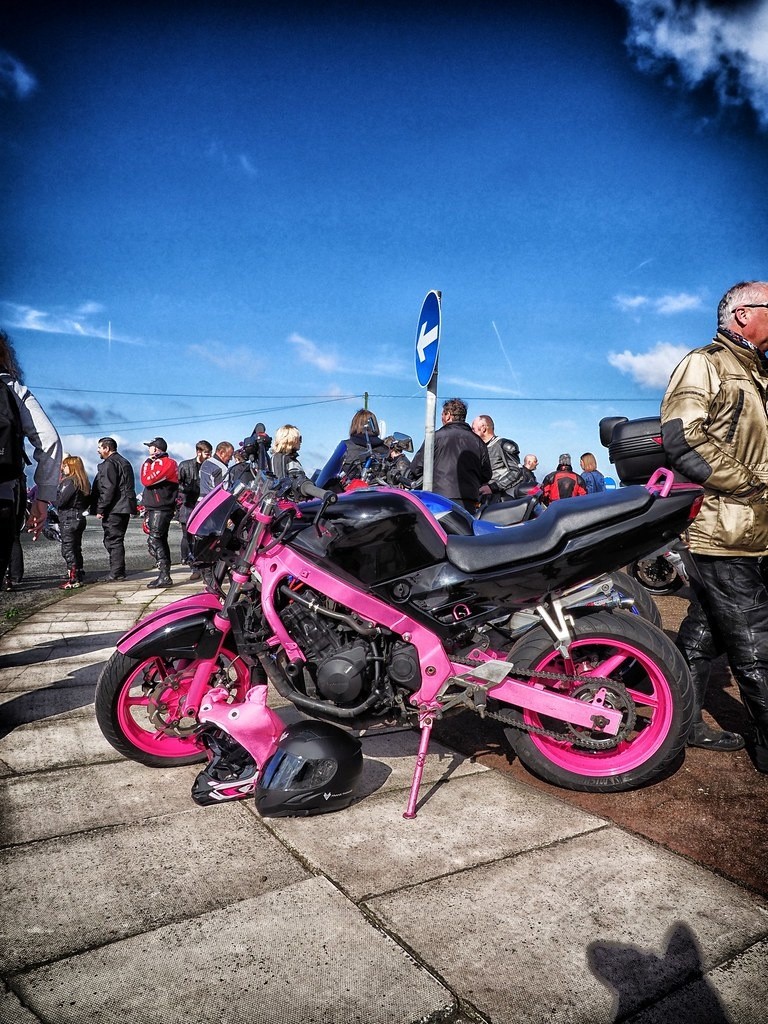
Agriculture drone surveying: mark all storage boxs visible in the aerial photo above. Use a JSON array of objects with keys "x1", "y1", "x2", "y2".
[{"x1": 609, "y1": 417, "x2": 674, "y2": 486}]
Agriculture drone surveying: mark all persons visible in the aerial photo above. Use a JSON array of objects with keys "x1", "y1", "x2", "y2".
[
  {"x1": 271, "y1": 425, "x2": 305, "y2": 481},
  {"x1": 580, "y1": 452, "x2": 606, "y2": 494},
  {"x1": 543, "y1": 453, "x2": 587, "y2": 505},
  {"x1": 51, "y1": 456, "x2": 91, "y2": 589},
  {"x1": 178, "y1": 440, "x2": 258, "y2": 579},
  {"x1": 141, "y1": 437, "x2": 178, "y2": 588},
  {"x1": 660, "y1": 280, "x2": 768, "y2": 771},
  {"x1": 315, "y1": 408, "x2": 411, "y2": 490},
  {"x1": 472, "y1": 415, "x2": 539, "y2": 513},
  {"x1": 411, "y1": 397, "x2": 492, "y2": 517},
  {"x1": 90, "y1": 437, "x2": 137, "y2": 582},
  {"x1": 0, "y1": 331, "x2": 63, "y2": 591}
]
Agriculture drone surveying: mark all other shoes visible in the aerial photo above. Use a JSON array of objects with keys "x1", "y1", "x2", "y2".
[
  {"x1": 0, "y1": 579, "x2": 13, "y2": 591},
  {"x1": 190, "y1": 572, "x2": 201, "y2": 580},
  {"x1": 12, "y1": 578, "x2": 23, "y2": 585},
  {"x1": 93, "y1": 574, "x2": 126, "y2": 582}
]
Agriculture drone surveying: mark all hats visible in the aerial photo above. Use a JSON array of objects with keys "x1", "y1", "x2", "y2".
[{"x1": 143, "y1": 437, "x2": 167, "y2": 452}]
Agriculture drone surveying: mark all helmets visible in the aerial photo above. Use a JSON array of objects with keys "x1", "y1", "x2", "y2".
[
  {"x1": 191, "y1": 685, "x2": 287, "y2": 806},
  {"x1": 254, "y1": 719, "x2": 364, "y2": 817}
]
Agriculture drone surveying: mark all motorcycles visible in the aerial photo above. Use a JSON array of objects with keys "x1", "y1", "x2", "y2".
[
  {"x1": 94, "y1": 415, "x2": 707, "y2": 815},
  {"x1": 20, "y1": 487, "x2": 62, "y2": 543}
]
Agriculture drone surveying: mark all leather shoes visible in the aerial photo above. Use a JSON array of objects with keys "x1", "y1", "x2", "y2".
[{"x1": 686, "y1": 723, "x2": 745, "y2": 751}]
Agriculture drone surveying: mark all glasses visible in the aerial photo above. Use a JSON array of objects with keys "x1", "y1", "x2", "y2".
[{"x1": 299, "y1": 435, "x2": 303, "y2": 443}]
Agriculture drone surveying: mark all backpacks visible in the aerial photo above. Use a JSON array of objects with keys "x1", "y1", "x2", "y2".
[{"x1": 0, "y1": 375, "x2": 33, "y2": 483}]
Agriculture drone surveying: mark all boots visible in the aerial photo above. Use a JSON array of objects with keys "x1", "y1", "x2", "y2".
[
  {"x1": 147, "y1": 571, "x2": 173, "y2": 588},
  {"x1": 58, "y1": 566, "x2": 83, "y2": 589}
]
